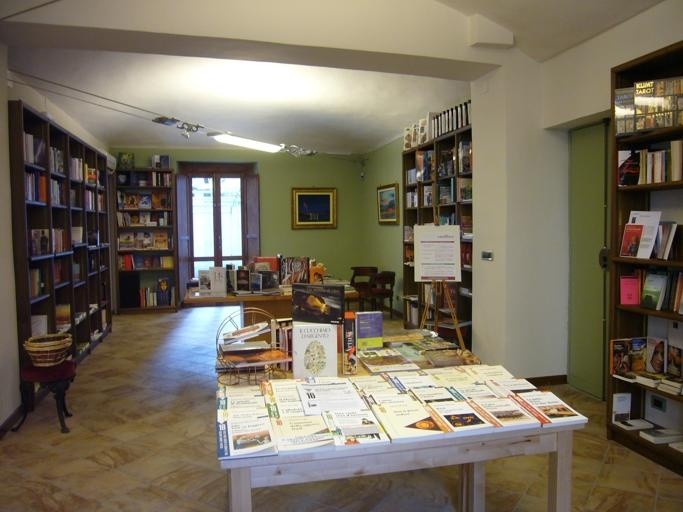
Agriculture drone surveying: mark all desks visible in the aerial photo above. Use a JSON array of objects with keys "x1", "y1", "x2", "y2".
[
  {"x1": 217, "y1": 329, "x2": 584, "y2": 511},
  {"x1": 180, "y1": 274, "x2": 358, "y2": 344}
]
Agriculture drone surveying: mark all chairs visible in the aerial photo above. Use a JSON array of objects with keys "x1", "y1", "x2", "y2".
[{"x1": 347, "y1": 266, "x2": 395, "y2": 320}]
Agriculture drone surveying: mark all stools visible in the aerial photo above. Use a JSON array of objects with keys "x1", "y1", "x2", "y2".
[{"x1": 10, "y1": 360, "x2": 75, "y2": 434}]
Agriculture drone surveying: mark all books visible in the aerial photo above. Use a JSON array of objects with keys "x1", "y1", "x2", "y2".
[{"x1": 20, "y1": 124, "x2": 175, "y2": 374}]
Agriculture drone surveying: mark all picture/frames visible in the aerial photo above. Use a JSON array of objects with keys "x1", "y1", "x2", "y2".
[
  {"x1": 377, "y1": 182, "x2": 399, "y2": 226},
  {"x1": 290, "y1": 186, "x2": 338, "y2": 231}
]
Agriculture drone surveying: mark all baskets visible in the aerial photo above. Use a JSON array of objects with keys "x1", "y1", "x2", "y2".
[{"x1": 21, "y1": 331, "x2": 73, "y2": 368}]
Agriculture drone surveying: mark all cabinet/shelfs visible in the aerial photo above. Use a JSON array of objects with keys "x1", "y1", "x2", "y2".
[
  {"x1": 605, "y1": 40, "x2": 682, "y2": 473},
  {"x1": 110, "y1": 167, "x2": 179, "y2": 314},
  {"x1": 6, "y1": 99, "x2": 112, "y2": 411},
  {"x1": 401, "y1": 124, "x2": 472, "y2": 352}
]
